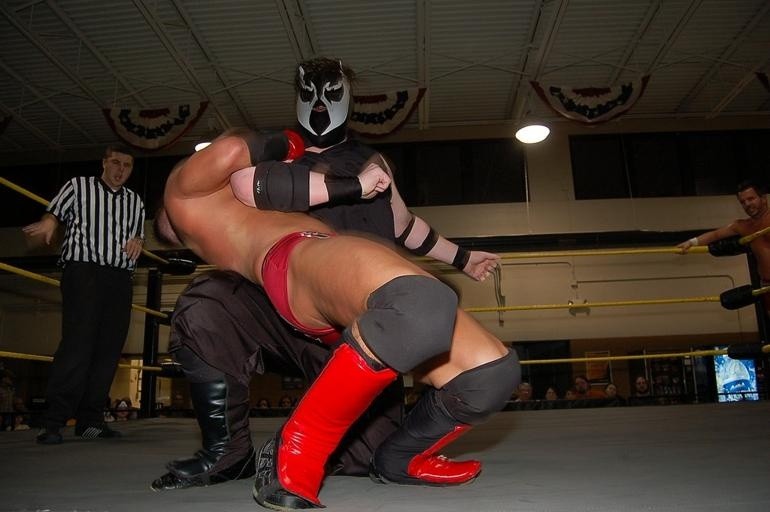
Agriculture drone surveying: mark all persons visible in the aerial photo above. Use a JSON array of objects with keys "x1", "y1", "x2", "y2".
[
  {"x1": 150, "y1": 56, "x2": 503, "y2": 493},
  {"x1": 21, "y1": 144, "x2": 145, "y2": 444},
  {"x1": 673, "y1": 182, "x2": 770, "y2": 323},
  {"x1": 152, "y1": 127, "x2": 521, "y2": 510}
]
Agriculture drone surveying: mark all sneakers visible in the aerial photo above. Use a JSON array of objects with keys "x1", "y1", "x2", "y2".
[
  {"x1": 73, "y1": 422, "x2": 122, "y2": 439},
  {"x1": 35, "y1": 431, "x2": 66, "y2": 444}
]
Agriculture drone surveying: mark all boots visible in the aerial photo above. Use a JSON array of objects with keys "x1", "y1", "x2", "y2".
[
  {"x1": 254, "y1": 329, "x2": 402, "y2": 510},
  {"x1": 151, "y1": 374, "x2": 257, "y2": 494},
  {"x1": 368, "y1": 388, "x2": 482, "y2": 489}
]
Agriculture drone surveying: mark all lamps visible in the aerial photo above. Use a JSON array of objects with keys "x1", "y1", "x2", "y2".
[{"x1": 509, "y1": 93, "x2": 552, "y2": 145}]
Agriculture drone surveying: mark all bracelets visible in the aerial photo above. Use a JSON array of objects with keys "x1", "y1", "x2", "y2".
[
  {"x1": 447, "y1": 241, "x2": 475, "y2": 271},
  {"x1": 322, "y1": 169, "x2": 363, "y2": 208},
  {"x1": 688, "y1": 236, "x2": 700, "y2": 247},
  {"x1": 133, "y1": 236, "x2": 144, "y2": 247}
]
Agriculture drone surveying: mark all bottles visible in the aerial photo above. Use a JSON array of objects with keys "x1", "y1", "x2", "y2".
[{"x1": 719, "y1": 349, "x2": 752, "y2": 402}]
